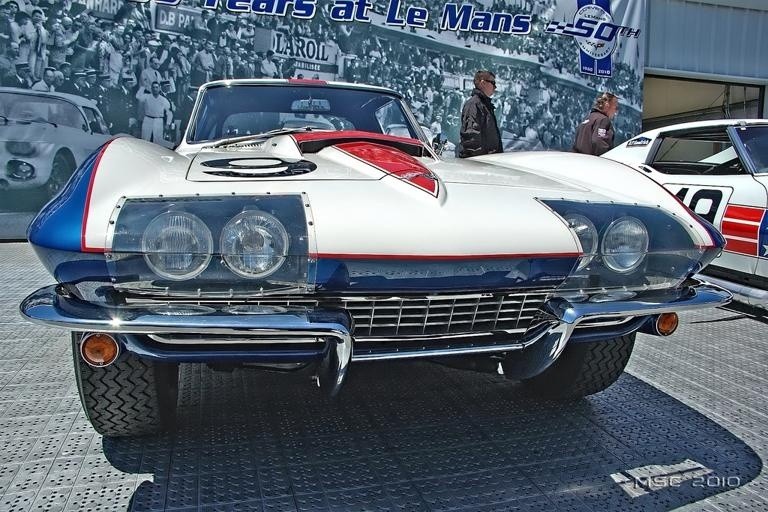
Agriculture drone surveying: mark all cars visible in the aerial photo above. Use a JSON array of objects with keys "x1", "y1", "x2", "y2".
[
  {"x1": 595, "y1": 118, "x2": 766, "y2": 312},
  {"x1": 19, "y1": 75, "x2": 728, "y2": 438},
  {"x1": 384, "y1": 124, "x2": 456, "y2": 160},
  {"x1": 1, "y1": 87, "x2": 118, "y2": 199}
]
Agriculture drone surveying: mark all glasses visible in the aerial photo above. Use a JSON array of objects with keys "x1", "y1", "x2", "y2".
[{"x1": 486, "y1": 79, "x2": 497, "y2": 88}]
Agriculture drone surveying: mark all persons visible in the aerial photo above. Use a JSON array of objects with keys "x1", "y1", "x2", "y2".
[{"x1": 0, "y1": 1, "x2": 643, "y2": 157}]
